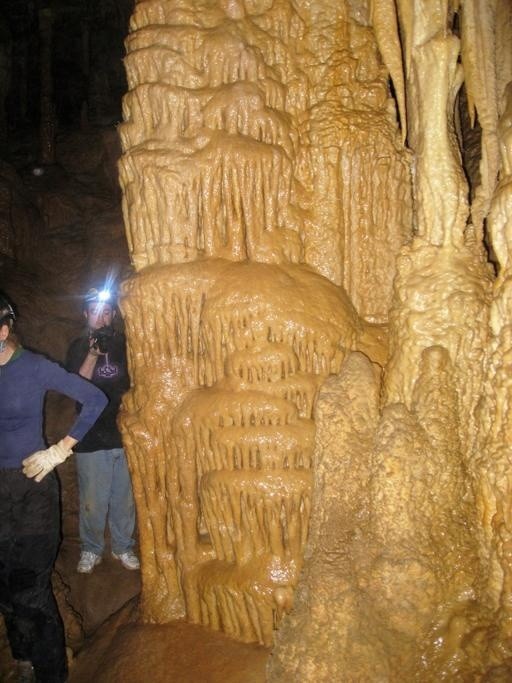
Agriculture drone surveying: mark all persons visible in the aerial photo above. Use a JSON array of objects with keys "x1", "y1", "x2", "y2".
[
  {"x1": 0, "y1": 291, "x2": 115, "y2": 682},
  {"x1": 64, "y1": 285, "x2": 143, "y2": 576}
]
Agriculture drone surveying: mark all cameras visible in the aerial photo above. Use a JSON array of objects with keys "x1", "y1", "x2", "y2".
[{"x1": 91, "y1": 325, "x2": 114, "y2": 353}]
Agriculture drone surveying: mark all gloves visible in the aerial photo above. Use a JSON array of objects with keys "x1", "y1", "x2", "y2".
[{"x1": 21, "y1": 436, "x2": 75, "y2": 483}]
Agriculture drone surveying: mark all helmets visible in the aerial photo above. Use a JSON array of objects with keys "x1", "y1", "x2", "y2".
[{"x1": 83, "y1": 284, "x2": 117, "y2": 314}]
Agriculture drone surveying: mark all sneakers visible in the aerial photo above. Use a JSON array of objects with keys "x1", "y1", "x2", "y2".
[
  {"x1": 111, "y1": 545, "x2": 140, "y2": 572},
  {"x1": 76, "y1": 550, "x2": 103, "y2": 573}
]
